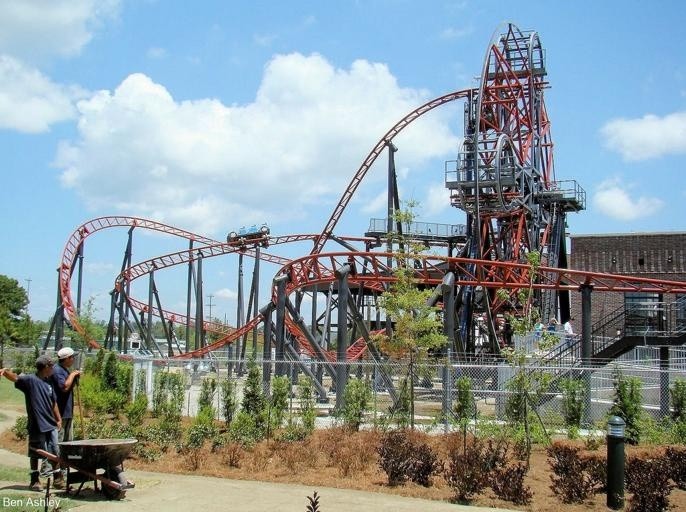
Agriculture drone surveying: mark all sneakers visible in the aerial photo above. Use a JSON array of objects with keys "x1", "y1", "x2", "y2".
[
  {"x1": 29, "y1": 480, "x2": 43, "y2": 491},
  {"x1": 52, "y1": 478, "x2": 73, "y2": 490}
]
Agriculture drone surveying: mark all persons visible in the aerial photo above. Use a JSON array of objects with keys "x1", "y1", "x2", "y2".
[
  {"x1": 532, "y1": 317, "x2": 578, "y2": 361},
  {"x1": 0, "y1": 354, "x2": 70, "y2": 492},
  {"x1": 39, "y1": 346, "x2": 81, "y2": 477}
]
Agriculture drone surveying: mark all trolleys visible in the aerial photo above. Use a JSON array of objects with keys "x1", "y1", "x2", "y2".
[{"x1": 29, "y1": 437, "x2": 140, "y2": 500}]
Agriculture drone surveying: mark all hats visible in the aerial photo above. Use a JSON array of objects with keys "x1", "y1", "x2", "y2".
[
  {"x1": 57, "y1": 346, "x2": 80, "y2": 359},
  {"x1": 34, "y1": 354, "x2": 56, "y2": 369}
]
